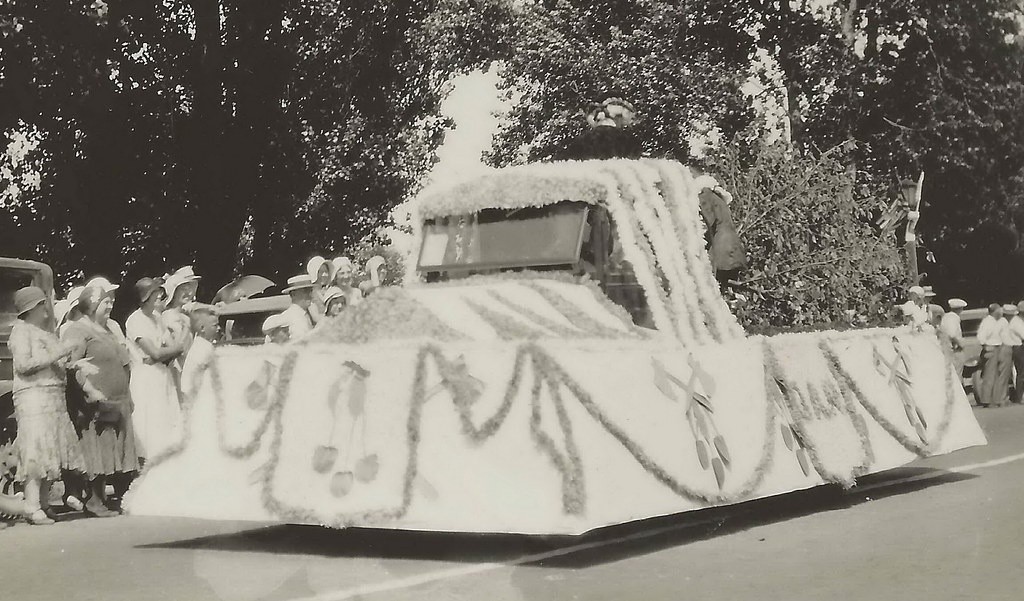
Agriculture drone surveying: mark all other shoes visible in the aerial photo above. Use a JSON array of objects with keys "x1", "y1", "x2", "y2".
[{"x1": 17, "y1": 489, "x2": 122, "y2": 525}]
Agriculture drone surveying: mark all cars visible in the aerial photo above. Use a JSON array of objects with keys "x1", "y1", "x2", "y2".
[
  {"x1": 211, "y1": 295, "x2": 302, "y2": 348},
  {"x1": 0, "y1": 257, "x2": 57, "y2": 428},
  {"x1": 950, "y1": 307, "x2": 998, "y2": 382}
]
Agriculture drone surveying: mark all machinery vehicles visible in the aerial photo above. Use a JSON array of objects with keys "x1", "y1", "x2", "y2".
[{"x1": 133, "y1": 156, "x2": 955, "y2": 536}]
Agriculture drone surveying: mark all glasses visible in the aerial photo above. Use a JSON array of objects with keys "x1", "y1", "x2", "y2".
[
  {"x1": 156, "y1": 295, "x2": 165, "y2": 301},
  {"x1": 39, "y1": 300, "x2": 48, "y2": 305}
]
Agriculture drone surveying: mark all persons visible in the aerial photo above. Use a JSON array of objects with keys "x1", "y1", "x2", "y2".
[
  {"x1": 694, "y1": 175, "x2": 748, "y2": 296},
  {"x1": 7, "y1": 253, "x2": 390, "y2": 527},
  {"x1": 898, "y1": 283, "x2": 1024, "y2": 406}
]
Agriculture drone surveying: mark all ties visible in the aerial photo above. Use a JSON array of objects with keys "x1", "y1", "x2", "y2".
[{"x1": 307, "y1": 309, "x2": 316, "y2": 326}]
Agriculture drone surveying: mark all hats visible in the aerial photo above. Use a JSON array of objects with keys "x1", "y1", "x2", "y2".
[
  {"x1": 135, "y1": 277, "x2": 166, "y2": 302},
  {"x1": 164, "y1": 275, "x2": 194, "y2": 307},
  {"x1": 949, "y1": 298, "x2": 967, "y2": 308},
  {"x1": 262, "y1": 314, "x2": 289, "y2": 335},
  {"x1": 923, "y1": 285, "x2": 936, "y2": 297},
  {"x1": 331, "y1": 257, "x2": 351, "y2": 281},
  {"x1": 14, "y1": 286, "x2": 46, "y2": 318},
  {"x1": 323, "y1": 286, "x2": 344, "y2": 314},
  {"x1": 1000, "y1": 304, "x2": 1019, "y2": 316},
  {"x1": 365, "y1": 255, "x2": 386, "y2": 271},
  {"x1": 174, "y1": 265, "x2": 202, "y2": 280},
  {"x1": 52, "y1": 277, "x2": 120, "y2": 330},
  {"x1": 307, "y1": 256, "x2": 337, "y2": 283},
  {"x1": 281, "y1": 274, "x2": 314, "y2": 294}
]
